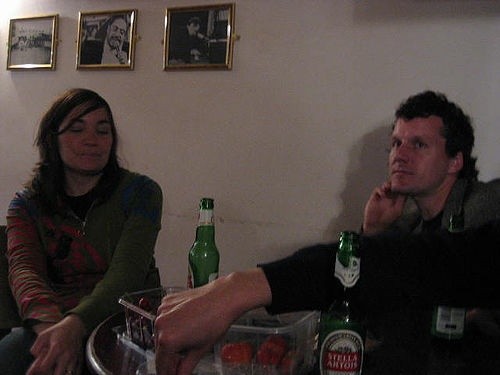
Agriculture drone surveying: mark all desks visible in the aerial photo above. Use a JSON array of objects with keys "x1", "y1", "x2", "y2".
[{"x1": 87, "y1": 309, "x2": 157, "y2": 375}]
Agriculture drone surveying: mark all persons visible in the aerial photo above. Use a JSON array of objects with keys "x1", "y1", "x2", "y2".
[
  {"x1": 0, "y1": 87, "x2": 162, "y2": 375},
  {"x1": 80, "y1": 16, "x2": 130, "y2": 65},
  {"x1": 151, "y1": 90, "x2": 500, "y2": 375},
  {"x1": 172, "y1": 17, "x2": 211, "y2": 64}
]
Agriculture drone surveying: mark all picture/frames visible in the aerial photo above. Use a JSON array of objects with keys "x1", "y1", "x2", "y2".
[
  {"x1": 6, "y1": 13, "x2": 59, "y2": 71},
  {"x1": 163, "y1": 3, "x2": 236, "y2": 71},
  {"x1": 76, "y1": 7, "x2": 138, "y2": 71}
]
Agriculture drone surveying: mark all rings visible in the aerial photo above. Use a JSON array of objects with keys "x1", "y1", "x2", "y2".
[{"x1": 66, "y1": 369, "x2": 73, "y2": 375}]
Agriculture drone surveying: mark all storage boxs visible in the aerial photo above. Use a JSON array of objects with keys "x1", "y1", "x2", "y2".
[{"x1": 214, "y1": 310, "x2": 320, "y2": 375}]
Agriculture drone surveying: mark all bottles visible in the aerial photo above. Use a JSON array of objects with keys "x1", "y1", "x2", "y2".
[
  {"x1": 316, "y1": 229, "x2": 365, "y2": 375},
  {"x1": 187, "y1": 198, "x2": 220, "y2": 288},
  {"x1": 432, "y1": 212, "x2": 467, "y2": 340}
]
